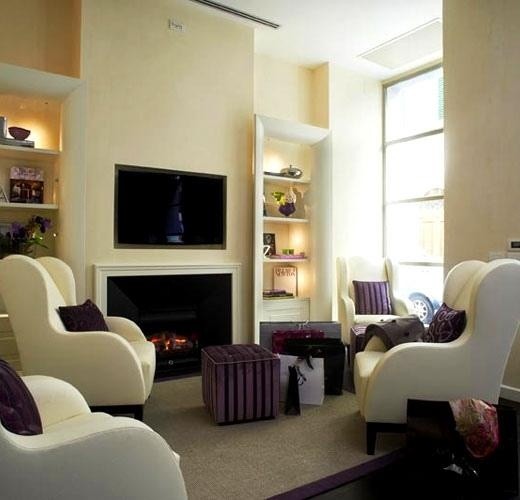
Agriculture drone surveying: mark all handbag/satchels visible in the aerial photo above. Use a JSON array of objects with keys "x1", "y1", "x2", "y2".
[{"x1": 272, "y1": 329, "x2": 345, "y2": 415}]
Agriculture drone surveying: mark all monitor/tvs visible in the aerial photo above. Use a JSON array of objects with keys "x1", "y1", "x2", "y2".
[{"x1": 114, "y1": 164, "x2": 227, "y2": 249}]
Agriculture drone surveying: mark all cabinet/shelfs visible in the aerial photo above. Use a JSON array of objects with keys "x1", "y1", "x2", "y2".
[
  {"x1": 0, "y1": 61, "x2": 93, "y2": 373},
  {"x1": 250, "y1": 110, "x2": 339, "y2": 352}
]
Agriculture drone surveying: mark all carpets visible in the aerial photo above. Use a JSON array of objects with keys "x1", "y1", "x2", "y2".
[{"x1": 127, "y1": 365, "x2": 431, "y2": 498}]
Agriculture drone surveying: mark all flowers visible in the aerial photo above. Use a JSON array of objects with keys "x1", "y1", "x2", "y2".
[{"x1": 1, "y1": 213, "x2": 59, "y2": 258}]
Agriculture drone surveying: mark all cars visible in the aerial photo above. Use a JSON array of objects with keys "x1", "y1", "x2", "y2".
[{"x1": 396, "y1": 275, "x2": 443, "y2": 324}]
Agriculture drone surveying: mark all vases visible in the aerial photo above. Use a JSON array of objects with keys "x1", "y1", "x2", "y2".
[{"x1": 14, "y1": 239, "x2": 38, "y2": 259}]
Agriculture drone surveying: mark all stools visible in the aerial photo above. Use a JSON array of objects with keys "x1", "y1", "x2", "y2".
[
  {"x1": 350, "y1": 326, "x2": 370, "y2": 364},
  {"x1": 199, "y1": 343, "x2": 283, "y2": 426}
]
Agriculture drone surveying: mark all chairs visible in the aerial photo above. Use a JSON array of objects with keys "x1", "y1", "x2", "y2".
[
  {"x1": 334, "y1": 253, "x2": 412, "y2": 367},
  {"x1": 0, "y1": 249, "x2": 159, "y2": 426},
  {"x1": 351, "y1": 254, "x2": 519, "y2": 459},
  {"x1": 1, "y1": 362, "x2": 195, "y2": 500}
]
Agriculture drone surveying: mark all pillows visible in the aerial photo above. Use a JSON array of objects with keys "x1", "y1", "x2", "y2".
[
  {"x1": 352, "y1": 279, "x2": 393, "y2": 316},
  {"x1": 55, "y1": 297, "x2": 109, "y2": 332},
  {"x1": 0, "y1": 356, "x2": 44, "y2": 434},
  {"x1": 422, "y1": 301, "x2": 467, "y2": 344}
]
